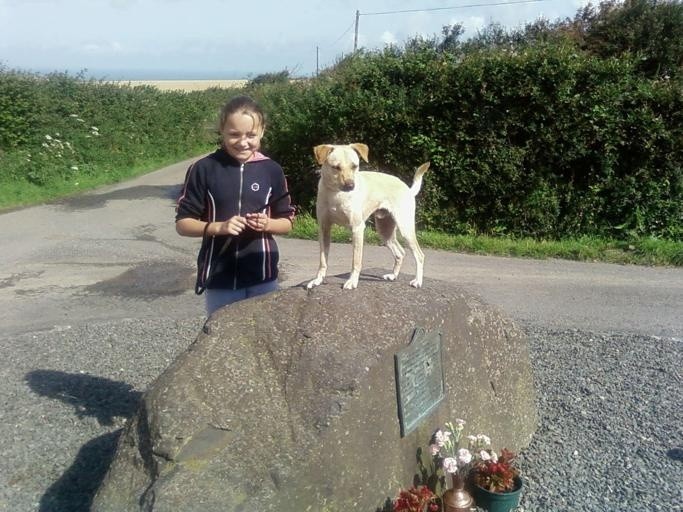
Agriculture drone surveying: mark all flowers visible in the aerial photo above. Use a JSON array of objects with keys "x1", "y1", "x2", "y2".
[
  {"x1": 374, "y1": 484, "x2": 438, "y2": 512},
  {"x1": 470, "y1": 447, "x2": 521, "y2": 493},
  {"x1": 428, "y1": 418, "x2": 498, "y2": 479}
]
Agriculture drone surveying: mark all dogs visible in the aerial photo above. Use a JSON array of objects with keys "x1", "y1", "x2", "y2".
[{"x1": 305, "y1": 142, "x2": 431, "y2": 292}]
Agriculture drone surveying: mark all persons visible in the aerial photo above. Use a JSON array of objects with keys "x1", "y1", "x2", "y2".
[{"x1": 174, "y1": 97, "x2": 295, "y2": 320}]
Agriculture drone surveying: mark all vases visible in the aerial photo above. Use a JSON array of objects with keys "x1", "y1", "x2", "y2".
[
  {"x1": 442, "y1": 477, "x2": 470, "y2": 512},
  {"x1": 473, "y1": 475, "x2": 523, "y2": 512}
]
